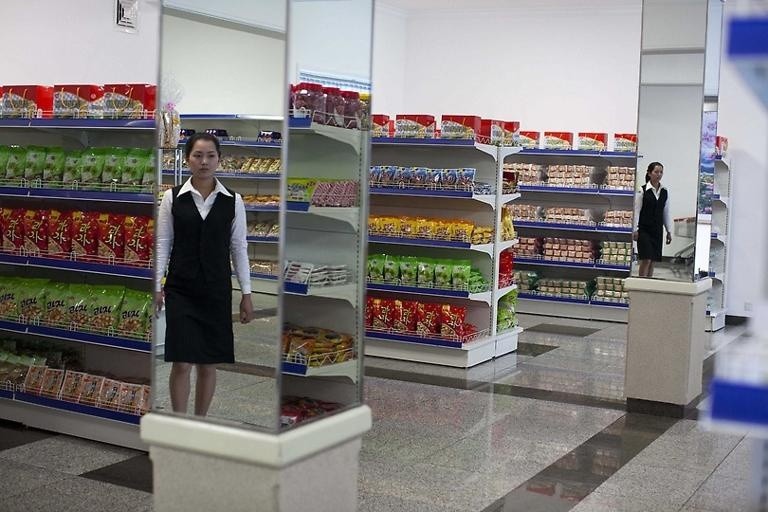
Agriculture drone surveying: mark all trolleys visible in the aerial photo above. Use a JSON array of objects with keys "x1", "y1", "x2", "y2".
[{"x1": 670, "y1": 217, "x2": 696, "y2": 267}]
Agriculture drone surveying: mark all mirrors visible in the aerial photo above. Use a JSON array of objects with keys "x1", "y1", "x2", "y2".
[
  {"x1": 630, "y1": 1, "x2": 722, "y2": 283},
  {"x1": 286, "y1": 0, "x2": 376, "y2": 436},
  {"x1": 145, "y1": 0, "x2": 287, "y2": 443}
]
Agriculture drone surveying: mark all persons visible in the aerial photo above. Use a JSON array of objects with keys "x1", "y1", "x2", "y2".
[
  {"x1": 632, "y1": 161, "x2": 672, "y2": 275},
  {"x1": 156, "y1": 132, "x2": 253, "y2": 417}
]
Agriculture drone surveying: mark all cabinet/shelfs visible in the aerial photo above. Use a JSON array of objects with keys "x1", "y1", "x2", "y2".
[
  {"x1": 504, "y1": 126, "x2": 731, "y2": 331},
  {"x1": 286, "y1": 105, "x2": 366, "y2": 388},
  {"x1": 1, "y1": 82, "x2": 157, "y2": 452},
  {"x1": 369, "y1": 112, "x2": 528, "y2": 373},
  {"x1": 157, "y1": 109, "x2": 284, "y2": 301}
]
